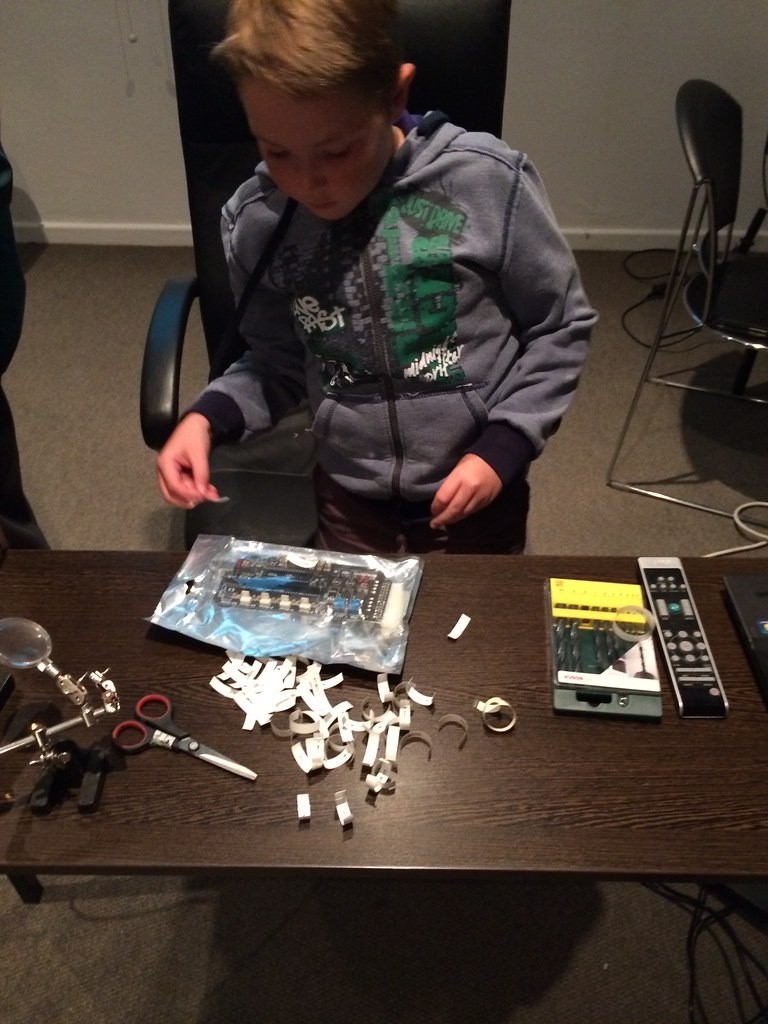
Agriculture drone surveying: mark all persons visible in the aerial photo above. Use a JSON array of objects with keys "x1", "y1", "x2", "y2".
[
  {"x1": 158, "y1": 1, "x2": 597, "y2": 556},
  {"x1": 0, "y1": 144, "x2": 51, "y2": 550}
]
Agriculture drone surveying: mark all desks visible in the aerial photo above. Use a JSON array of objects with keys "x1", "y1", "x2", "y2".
[{"x1": 0, "y1": 548, "x2": 767, "y2": 904}]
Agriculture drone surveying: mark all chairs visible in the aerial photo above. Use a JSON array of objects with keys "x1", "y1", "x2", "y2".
[
  {"x1": 603, "y1": 78, "x2": 768, "y2": 528},
  {"x1": 139, "y1": 1, "x2": 512, "y2": 553}
]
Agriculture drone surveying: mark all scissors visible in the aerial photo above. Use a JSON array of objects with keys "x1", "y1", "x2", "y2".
[{"x1": 111, "y1": 694, "x2": 259, "y2": 782}]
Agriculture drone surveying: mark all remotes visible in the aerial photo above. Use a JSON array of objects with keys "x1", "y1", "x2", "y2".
[{"x1": 638, "y1": 557, "x2": 729, "y2": 717}]
